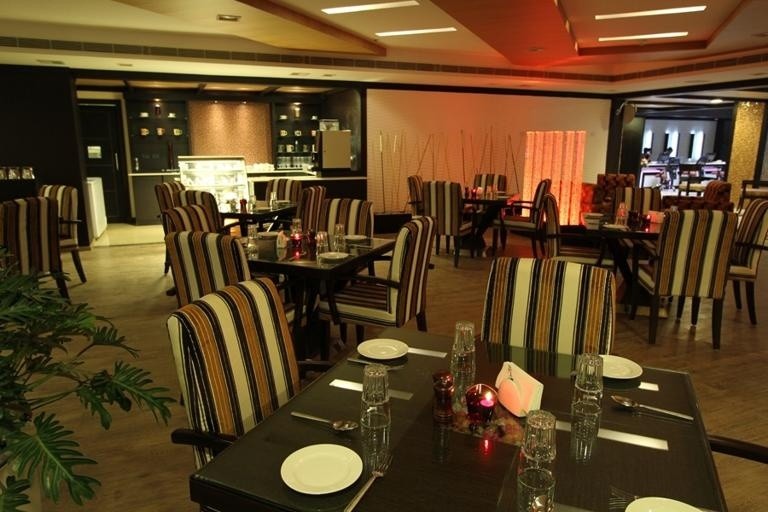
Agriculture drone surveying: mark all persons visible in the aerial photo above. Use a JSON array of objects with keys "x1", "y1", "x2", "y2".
[{"x1": 657, "y1": 148, "x2": 672, "y2": 162}]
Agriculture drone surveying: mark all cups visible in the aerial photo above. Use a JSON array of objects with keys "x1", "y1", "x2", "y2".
[
  {"x1": 334, "y1": 239, "x2": 346, "y2": 250},
  {"x1": 641, "y1": 214, "x2": 651, "y2": 224},
  {"x1": 649, "y1": 224, "x2": 661, "y2": 233},
  {"x1": 316, "y1": 246, "x2": 329, "y2": 257},
  {"x1": 569, "y1": 402, "x2": 601, "y2": 463},
  {"x1": 617, "y1": 203, "x2": 627, "y2": 217},
  {"x1": 276, "y1": 157, "x2": 311, "y2": 168},
  {"x1": 452, "y1": 369, "x2": 477, "y2": 411},
  {"x1": 333, "y1": 224, "x2": 345, "y2": 239},
  {"x1": 473, "y1": 428, "x2": 507, "y2": 472},
  {"x1": 628, "y1": 211, "x2": 638, "y2": 219},
  {"x1": 316, "y1": 231, "x2": 329, "y2": 247},
  {"x1": 615, "y1": 218, "x2": 626, "y2": 225},
  {"x1": 628, "y1": 219, "x2": 637, "y2": 226},
  {"x1": 0, "y1": 166, "x2": 35, "y2": 181},
  {"x1": 140, "y1": 111, "x2": 183, "y2": 136},
  {"x1": 359, "y1": 405, "x2": 392, "y2": 452},
  {"x1": 515, "y1": 466, "x2": 557, "y2": 512},
  {"x1": 277, "y1": 114, "x2": 316, "y2": 153},
  {"x1": 247, "y1": 163, "x2": 275, "y2": 173},
  {"x1": 464, "y1": 384, "x2": 496, "y2": 420},
  {"x1": 361, "y1": 364, "x2": 388, "y2": 405},
  {"x1": 247, "y1": 244, "x2": 258, "y2": 258},
  {"x1": 573, "y1": 353, "x2": 603, "y2": 394},
  {"x1": 571, "y1": 389, "x2": 603, "y2": 403},
  {"x1": 640, "y1": 223, "x2": 650, "y2": 232},
  {"x1": 522, "y1": 408, "x2": 556, "y2": 463},
  {"x1": 247, "y1": 224, "x2": 258, "y2": 240},
  {"x1": 649, "y1": 210, "x2": 664, "y2": 224},
  {"x1": 449, "y1": 319, "x2": 477, "y2": 373}
]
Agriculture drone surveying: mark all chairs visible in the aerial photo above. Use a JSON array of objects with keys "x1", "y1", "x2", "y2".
[
  {"x1": 631, "y1": 209, "x2": 736, "y2": 349},
  {"x1": 545, "y1": 173, "x2": 768, "y2": 326},
  {"x1": 404, "y1": 174, "x2": 554, "y2": 268},
  {"x1": 37, "y1": 183, "x2": 88, "y2": 285},
  {"x1": 155, "y1": 174, "x2": 438, "y2": 357},
  {"x1": 0, "y1": 195, "x2": 73, "y2": 306},
  {"x1": 164, "y1": 277, "x2": 350, "y2": 471},
  {"x1": 474, "y1": 255, "x2": 618, "y2": 360}
]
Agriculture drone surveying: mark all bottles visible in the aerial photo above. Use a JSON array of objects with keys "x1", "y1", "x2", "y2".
[
  {"x1": 230, "y1": 196, "x2": 257, "y2": 214},
  {"x1": 275, "y1": 249, "x2": 312, "y2": 260},
  {"x1": 464, "y1": 183, "x2": 498, "y2": 198},
  {"x1": 430, "y1": 422, "x2": 453, "y2": 467},
  {"x1": 270, "y1": 192, "x2": 277, "y2": 210},
  {"x1": 430, "y1": 372, "x2": 453, "y2": 420},
  {"x1": 275, "y1": 219, "x2": 315, "y2": 249}
]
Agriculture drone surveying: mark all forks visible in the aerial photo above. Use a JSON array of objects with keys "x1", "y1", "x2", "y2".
[
  {"x1": 609, "y1": 486, "x2": 717, "y2": 511},
  {"x1": 347, "y1": 356, "x2": 405, "y2": 372},
  {"x1": 609, "y1": 497, "x2": 630, "y2": 509},
  {"x1": 344, "y1": 454, "x2": 394, "y2": 512}
]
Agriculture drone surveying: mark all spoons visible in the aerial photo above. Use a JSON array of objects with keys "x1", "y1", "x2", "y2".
[
  {"x1": 290, "y1": 411, "x2": 358, "y2": 432},
  {"x1": 611, "y1": 394, "x2": 694, "y2": 421}
]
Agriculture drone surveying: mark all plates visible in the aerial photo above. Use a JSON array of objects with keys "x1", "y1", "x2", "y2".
[
  {"x1": 257, "y1": 208, "x2": 271, "y2": 211},
  {"x1": 344, "y1": 234, "x2": 368, "y2": 242},
  {"x1": 277, "y1": 201, "x2": 291, "y2": 205},
  {"x1": 280, "y1": 442, "x2": 363, "y2": 495},
  {"x1": 624, "y1": 496, "x2": 704, "y2": 511},
  {"x1": 603, "y1": 224, "x2": 627, "y2": 230},
  {"x1": 318, "y1": 251, "x2": 348, "y2": 260},
  {"x1": 357, "y1": 338, "x2": 409, "y2": 360},
  {"x1": 599, "y1": 355, "x2": 644, "y2": 380}
]
